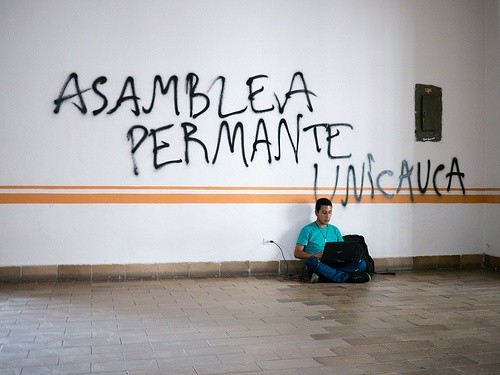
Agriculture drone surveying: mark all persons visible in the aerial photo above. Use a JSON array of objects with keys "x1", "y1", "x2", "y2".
[{"x1": 295, "y1": 198, "x2": 373, "y2": 283}]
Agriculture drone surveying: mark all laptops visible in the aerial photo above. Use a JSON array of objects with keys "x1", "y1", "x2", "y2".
[{"x1": 320, "y1": 241, "x2": 363, "y2": 267}]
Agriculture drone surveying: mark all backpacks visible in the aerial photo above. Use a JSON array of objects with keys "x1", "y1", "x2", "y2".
[{"x1": 342, "y1": 235, "x2": 396, "y2": 276}]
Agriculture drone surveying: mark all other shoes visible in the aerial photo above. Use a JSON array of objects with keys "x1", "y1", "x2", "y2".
[
  {"x1": 346, "y1": 272, "x2": 372, "y2": 283},
  {"x1": 310, "y1": 273, "x2": 320, "y2": 283}
]
